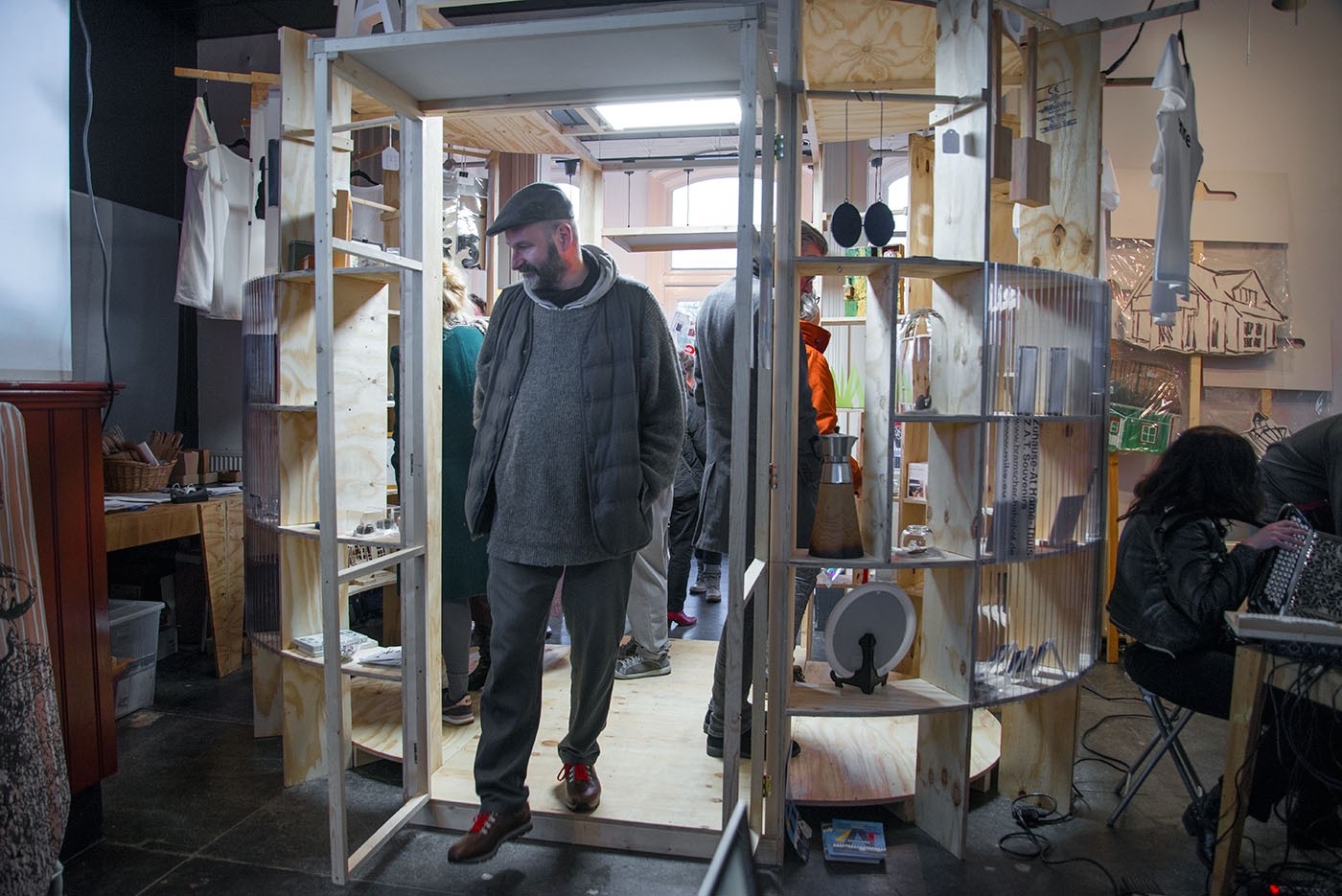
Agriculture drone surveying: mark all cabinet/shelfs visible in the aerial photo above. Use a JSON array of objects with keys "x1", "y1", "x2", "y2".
[
  {"x1": 0, "y1": 398, "x2": 116, "y2": 837},
  {"x1": 272, "y1": 116, "x2": 443, "y2": 787},
  {"x1": 779, "y1": 257, "x2": 1111, "y2": 808}
]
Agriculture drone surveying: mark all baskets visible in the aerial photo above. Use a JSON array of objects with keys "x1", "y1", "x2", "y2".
[{"x1": 104, "y1": 459, "x2": 178, "y2": 492}]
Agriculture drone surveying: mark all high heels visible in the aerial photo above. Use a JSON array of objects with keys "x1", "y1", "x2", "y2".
[{"x1": 668, "y1": 612, "x2": 697, "y2": 628}]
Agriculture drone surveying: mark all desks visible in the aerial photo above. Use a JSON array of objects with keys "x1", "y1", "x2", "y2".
[
  {"x1": 1207, "y1": 637, "x2": 1342, "y2": 896},
  {"x1": 103, "y1": 479, "x2": 246, "y2": 681}
]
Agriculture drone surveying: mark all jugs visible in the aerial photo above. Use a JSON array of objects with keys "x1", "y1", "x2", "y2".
[{"x1": 808, "y1": 427, "x2": 866, "y2": 559}]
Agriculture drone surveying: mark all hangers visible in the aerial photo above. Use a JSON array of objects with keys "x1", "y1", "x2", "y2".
[
  {"x1": 1173, "y1": 1, "x2": 1190, "y2": 73},
  {"x1": 203, "y1": 79, "x2": 211, "y2": 123},
  {"x1": 225, "y1": 123, "x2": 249, "y2": 149}
]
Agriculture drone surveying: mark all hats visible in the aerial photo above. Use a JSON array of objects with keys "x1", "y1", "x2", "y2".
[{"x1": 484, "y1": 183, "x2": 574, "y2": 236}]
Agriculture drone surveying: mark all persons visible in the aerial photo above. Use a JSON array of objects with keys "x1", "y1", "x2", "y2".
[
  {"x1": 393, "y1": 220, "x2": 859, "y2": 760},
  {"x1": 1105, "y1": 414, "x2": 1342, "y2": 881},
  {"x1": 450, "y1": 180, "x2": 684, "y2": 863}
]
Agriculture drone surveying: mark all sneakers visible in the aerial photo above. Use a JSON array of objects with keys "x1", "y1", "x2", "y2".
[
  {"x1": 617, "y1": 638, "x2": 673, "y2": 663},
  {"x1": 442, "y1": 688, "x2": 475, "y2": 727},
  {"x1": 614, "y1": 645, "x2": 671, "y2": 679},
  {"x1": 448, "y1": 801, "x2": 533, "y2": 865},
  {"x1": 557, "y1": 764, "x2": 602, "y2": 812},
  {"x1": 703, "y1": 709, "x2": 801, "y2": 759}
]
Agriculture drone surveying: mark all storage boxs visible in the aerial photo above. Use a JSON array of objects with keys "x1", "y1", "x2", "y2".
[{"x1": 109, "y1": 599, "x2": 167, "y2": 720}]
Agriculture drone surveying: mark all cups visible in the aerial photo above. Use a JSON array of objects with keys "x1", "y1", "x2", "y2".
[{"x1": 901, "y1": 525, "x2": 935, "y2": 554}]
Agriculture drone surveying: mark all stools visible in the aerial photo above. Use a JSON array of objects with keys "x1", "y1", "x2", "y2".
[{"x1": 1105, "y1": 670, "x2": 1199, "y2": 829}]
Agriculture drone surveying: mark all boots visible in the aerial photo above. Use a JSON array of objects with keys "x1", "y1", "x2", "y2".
[
  {"x1": 1182, "y1": 775, "x2": 1223, "y2": 871},
  {"x1": 705, "y1": 565, "x2": 721, "y2": 602},
  {"x1": 690, "y1": 559, "x2": 706, "y2": 592},
  {"x1": 467, "y1": 625, "x2": 491, "y2": 691}
]
